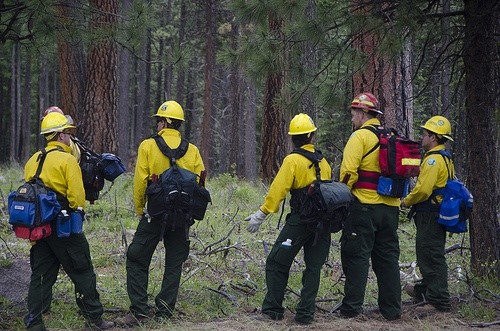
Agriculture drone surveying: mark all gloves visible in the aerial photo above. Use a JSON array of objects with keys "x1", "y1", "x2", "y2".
[{"x1": 244, "y1": 208, "x2": 268, "y2": 233}]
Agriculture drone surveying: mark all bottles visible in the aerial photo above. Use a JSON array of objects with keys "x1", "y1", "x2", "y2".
[
  {"x1": 282, "y1": 239, "x2": 292, "y2": 246},
  {"x1": 77, "y1": 207, "x2": 83, "y2": 211},
  {"x1": 58, "y1": 210, "x2": 69, "y2": 217}
]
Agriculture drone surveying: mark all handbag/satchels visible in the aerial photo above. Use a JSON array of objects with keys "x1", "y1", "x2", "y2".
[{"x1": 97, "y1": 153, "x2": 127, "y2": 199}]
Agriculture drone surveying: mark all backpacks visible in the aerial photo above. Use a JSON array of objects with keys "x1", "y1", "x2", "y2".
[
  {"x1": 357, "y1": 126, "x2": 422, "y2": 197},
  {"x1": 8, "y1": 147, "x2": 74, "y2": 240},
  {"x1": 70, "y1": 134, "x2": 104, "y2": 204},
  {"x1": 425, "y1": 149, "x2": 474, "y2": 238},
  {"x1": 290, "y1": 148, "x2": 353, "y2": 232},
  {"x1": 145, "y1": 133, "x2": 212, "y2": 240}
]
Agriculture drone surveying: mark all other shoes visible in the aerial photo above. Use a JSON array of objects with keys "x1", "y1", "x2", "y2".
[
  {"x1": 404, "y1": 283, "x2": 418, "y2": 298},
  {"x1": 427, "y1": 301, "x2": 450, "y2": 311},
  {"x1": 85, "y1": 318, "x2": 114, "y2": 329}
]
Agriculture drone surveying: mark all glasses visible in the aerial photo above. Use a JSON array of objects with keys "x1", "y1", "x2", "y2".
[{"x1": 420, "y1": 132, "x2": 433, "y2": 137}]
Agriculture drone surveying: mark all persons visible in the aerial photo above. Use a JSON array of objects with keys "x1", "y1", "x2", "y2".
[
  {"x1": 115, "y1": 100, "x2": 206, "y2": 325},
  {"x1": 244, "y1": 113, "x2": 332, "y2": 325},
  {"x1": 335, "y1": 92, "x2": 402, "y2": 321},
  {"x1": 23, "y1": 106, "x2": 115, "y2": 331},
  {"x1": 399, "y1": 115, "x2": 455, "y2": 314}
]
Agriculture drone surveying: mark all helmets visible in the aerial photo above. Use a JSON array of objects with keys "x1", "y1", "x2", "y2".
[
  {"x1": 153, "y1": 101, "x2": 186, "y2": 122},
  {"x1": 40, "y1": 106, "x2": 77, "y2": 134},
  {"x1": 419, "y1": 115, "x2": 457, "y2": 144},
  {"x1": 288, "y1": 113, "x2": 317, "y2": 135},
  {"x1": 347, "y1": 92, "x2": 384, "y2": 115}
]
